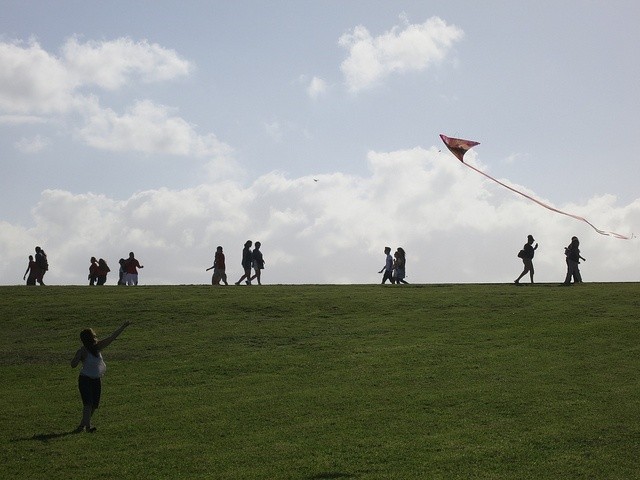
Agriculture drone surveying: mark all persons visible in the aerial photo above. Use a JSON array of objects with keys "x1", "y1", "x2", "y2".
[
  {"x1": 123, "y1": 252, "x2": 144, "y2": 286},
  {"x1": 96, "y1": 258, "x2": 110, "y2": 286},
  {"x1": 70, "y1": 320, "x2": 133, "y2": 434},
  {"x1": 514, "y1": 235, "x2": 539, "y2": 286},
  {"x1": 235, "y1": 240, "x2": 252, "y2": 286},
  {"x1": 87, "y1": 257, "x2": 98, "y2": 286},
  {"x1": 215, "y1": 246, "x2": 228, "y2": 286},
  {"x1": 245, "y1": 241, "x2": 265, "y2": 285},
  {"x1": 206, "y1": 252, "x2": 221, "y2": 285},
  {"x1": 378, "y1": 247, "x2": 394, "y2": 285},
  {"x1": 118, "y1": 258, "x2": 126, "y2": 285},
  {"x1": 392, "y1": 247, "x2": 409, "y2": 285},
  {"x1": 22, "y1": 246, "x2": 49, "y2": 286},
  {"x1": 562, "y1": 236, "x2": 586, "y2": 284},
  {"x1": 391, "y1": 252, "x2": 400, "y2": 284}
]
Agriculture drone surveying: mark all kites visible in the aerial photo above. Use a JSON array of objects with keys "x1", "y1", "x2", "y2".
[{"x1": 440, "y1": 134, "x2": 637, "y2": 241}]
